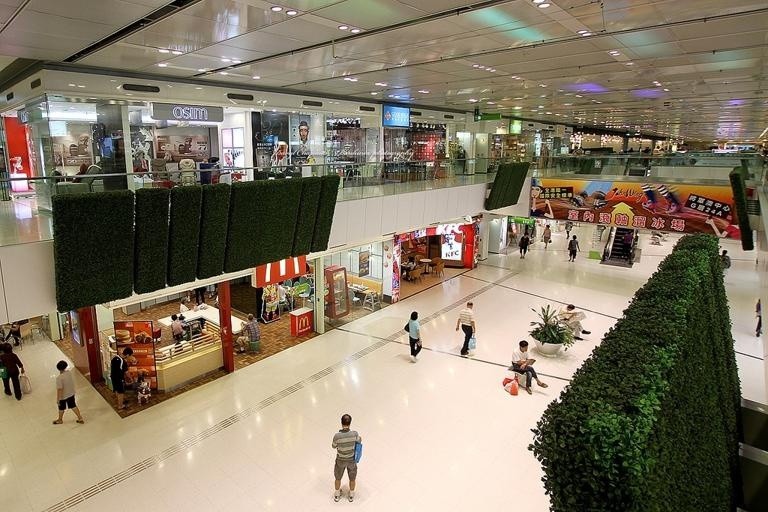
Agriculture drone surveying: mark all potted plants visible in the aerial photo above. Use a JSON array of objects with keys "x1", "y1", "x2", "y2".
[{"x1": 528, "y1": 302, "x2": 576, "y2": 359}]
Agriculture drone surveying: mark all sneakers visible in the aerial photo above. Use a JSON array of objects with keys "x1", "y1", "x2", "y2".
[
  {"x1": 526, "y1": 387, "x2": 532, "y2": 394},
  {"x1": 349, "y1": 496, "x2": 353, "y2": 501},
  {"x1": 334, "y1": 489, "x2": 342, "y2": 501},
  {"x1": 537, "y1": 382, "x2": 548, "y2": 388}
]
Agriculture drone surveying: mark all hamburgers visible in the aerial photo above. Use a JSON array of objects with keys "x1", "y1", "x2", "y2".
[{"x1": 116, "y1": 330, "x2": 131, "y2": 346}]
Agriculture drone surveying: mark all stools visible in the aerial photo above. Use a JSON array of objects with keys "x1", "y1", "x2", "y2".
[
  {"x1": 152, "y1": 326, "x2": 162, "y2": 343},
  {"x1": 513, "y1": 373, "x2": 525, "y2": 388},
  {"x1": 249, "y1": 340, "x2": 259, "y2": 351}
]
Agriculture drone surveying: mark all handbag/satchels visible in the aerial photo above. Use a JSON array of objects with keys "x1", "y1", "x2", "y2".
[
  {"x1": 469, "y1": 338, "x2": 477, "y2": 349},
  {"x1": 19, "y1": 376, "x2": 32, "y2": 394},
  {"x1": 0, "y1": 367, "x2": 7, "y2": 379},
  {"x1": 503, "y1": 378, "x2": 519, "y2": 395},
  {"x1": 354, "y1": 443, "x2": 362, "y2": 463},
  {"x1": 404, "y1": 323, "x2": 409, "y2": 332}
]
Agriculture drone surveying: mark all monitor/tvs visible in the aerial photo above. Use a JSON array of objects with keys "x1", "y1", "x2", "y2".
[
  {"x1": 382, "y1": 103, "x2": 410, "y2": 128},
  {"x1": 509, "y1": 117, "x2": 522, "y2": 136}
]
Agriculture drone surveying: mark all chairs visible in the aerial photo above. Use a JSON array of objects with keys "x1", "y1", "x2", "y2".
[
  {"x1": 7, "y1": 315, "x2": 46, "y2": 351},
  {"x1": 53, "y1": 165, "x2": 102, "y2": 194},
  {"x1": 179, "y1": 158, "x2": 197, "y2": 185},
  {"x1": 401, "y1": 253, "x2": 443, "y2": 284},
  {"x1": 150, "y1": 157, "x2": 173, "y2": 180},
  {"x1": 279, "y1": 274, "x2": 382, "y2": 313}
]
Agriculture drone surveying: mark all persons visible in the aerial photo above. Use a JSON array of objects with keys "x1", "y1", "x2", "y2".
[
  {"x1": 170, "y1": 287, "x2": 205, "y2": 344},
  {"x1": 52, "y1": 360, "x2": 84, "y2": 424},
  {"x1": 71, "y1": 163, "x2": 87, "y2": 183},
  {"x1": 572, "y1": 182, "x2": 613, "y2": 209},
  {"x1": 278, "y1": 281, "x2": 289, "y2": 310},
  {"x1": 720, "y1": 250, "x2": 731, "y2": 274},
  {"x1": 558, "y1": 304, "x2": 591, "y2": 341},
  {"x1": 456, "y1": 301, "x2": 475, "y2": 356},
  {"x1": 755, "y1": 298, "x2": 763, "y2": 338},
  {"x1": 541, "y1": 224, "x2": 551, "y2": 249},
  {"x1": 620, "y1": 231, "x2": 633, "y2": 263},
  {"x1": 511, "y1": 340, "x2": 548, "y2": 394},
  {"x1": 0, "y1": 322, "x2": 25, "y2": 401},
  {"x1": 518, "y1": 232, "x2": 530, "y2": 259},
  {"x1": 405, "y1": 255, "x2": 415, "y2": 280},
  {"x1": 565, "y1": 222, "x2": 572, "y2": 239},
  {"x1": 110, "y1": 347, "x2": 133, "y2": 410},
  {"x1": 236, "y1": 313, "x2": 260, "y2": 354},
  {"x1": 332, "y1": 413, "x2": 362, "y2": 502},
  {"x1": 705, "y1": 217, "x2": 742, "y2": 241},
  {"x1": 291, "y1": 121, "x2": 313, "y2": 153},
  {"x1": 407, "y1": 311, "x2": 422, "y2": 364},
  {"x1": 640, "y1": 183, "x2": 680, "y2": 214},
  {"x1": 567, "y1": 234, "x2": 581, "y2": 262}
]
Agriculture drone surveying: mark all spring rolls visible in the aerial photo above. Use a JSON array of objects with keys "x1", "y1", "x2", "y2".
[{"x1": 134, "y1": 331, "x2": 152, "y2": 344}]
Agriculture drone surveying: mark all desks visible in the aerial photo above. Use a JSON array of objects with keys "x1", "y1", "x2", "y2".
[{"x1": 158, "y1": 303, "x2": 250, "y2": 349}]
